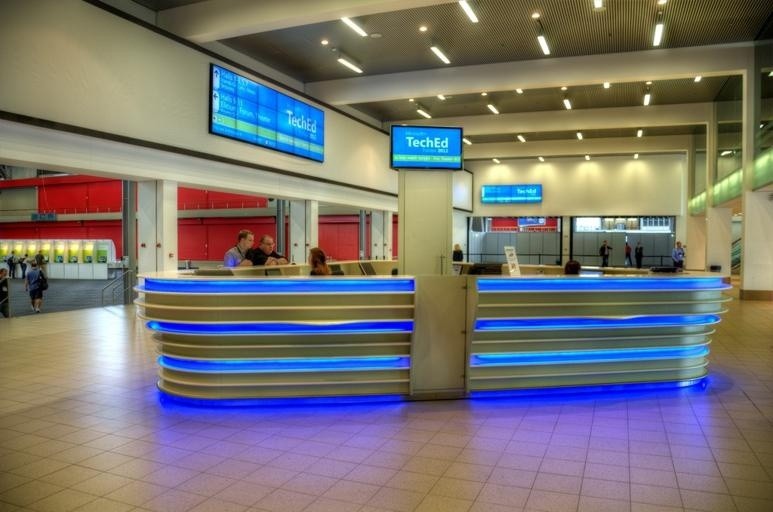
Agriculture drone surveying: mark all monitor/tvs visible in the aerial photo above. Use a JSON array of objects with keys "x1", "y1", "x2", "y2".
[
  {"x1": 266, "y1": 269, "x2": 282, "y2": 276},
  {"x1": 452, "y1": 265, "x2": 463, "y2": 275},
  {"x1": 578, "y1": 269, "x2": 605, "y2": 275},
  {"x1": 358, "y1": 261, "x2": 375, "y2": 275},
  {"x1": 0, "y1": 255, "x2": 106, "y2": 262},
  {"x1": 391, "y1": 124, "x2": 463, "y2": 170}
]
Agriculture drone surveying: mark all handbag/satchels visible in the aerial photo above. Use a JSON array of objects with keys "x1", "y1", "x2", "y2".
[{"x1": 36, "y1": 270, "x2": 48, "y2": 291}]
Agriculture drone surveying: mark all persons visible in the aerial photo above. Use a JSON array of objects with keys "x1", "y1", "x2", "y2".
[
  {"x1": 671, "y1": 242, "x2": 684, "y2": 267},
  {"x1": 0, "y1": 250, "x2": 44, "y2": 318},
  {"x1": 453, "y1": 244, "x2": 463, "y2": 261},
  {"x1": 625, "y1": 242, "x2": 632, "y2": 267},
  {"x1": 224, "y1": 229, "x2": 254, "y2": 267},
  {"x1": 308, "y1": 247, "x2": 332, "y2": 275},
  {"x1": 599, "y1": 240, "x2": 613, "y2": 267},
  {"x1": 635, "y1": 240, "x2": 643, "y2": 268},
  {"x1": 249, "y1": 234, "x2": 289, "y2": 265},
  {"x1": 565, "y1": 260, "x2": 581, "y2": 274}
]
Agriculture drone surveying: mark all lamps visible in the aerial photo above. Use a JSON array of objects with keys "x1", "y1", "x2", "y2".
[
  {"x1": 562, "y1": 93, "x2": 571, "y2": 109},
  {"x1": 486, "y1": 96, "x2": 500, "y2": 115},
  {"x1": 642, "y1": 85, "x2": 651, "y2": 106},
  {"x1": 332, "y1": 48, "x2": 363, "y2": 74},
  {"x1": 537, "y1": 18, "x2": 550, "y2": 55},
  {"x1": 429, "y1": 38, "x2": 451, "y2": 65},
  {"x1": 652, "y1": 10, "x2": 664, "y2": 47},
  {"x1": 414, "y1": 103, "x2": 431, "y2": 118}
]
